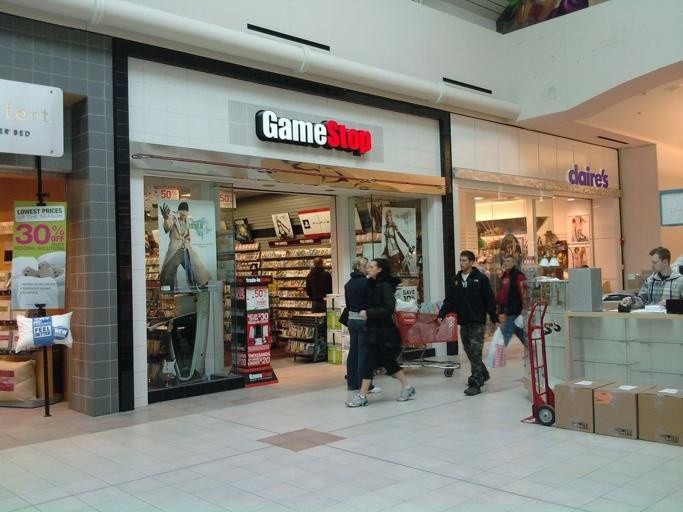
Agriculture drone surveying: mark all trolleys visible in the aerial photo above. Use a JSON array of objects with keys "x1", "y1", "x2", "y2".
[
  {"x1": 521, "y1": 298, "x2": 564, "y2": 428},
  {"x1": 379, "y1": 303, "x2": 463, "y2": 378}
]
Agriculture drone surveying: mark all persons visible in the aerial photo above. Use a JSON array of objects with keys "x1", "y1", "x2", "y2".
[
  {"x1": 345, "y1": 258, "x2": 415, "y2": 407},
  {"x1": 495, "y1": 255, "x2": 530, "y2": 348},
  {"x1": 569, "y1": 247, "x2": 585, "y2": 267},
  {"x1": 620, "y1": 246, "x2": 683, "y2": 307},
  {"x1": 307, "y1": 258, "x2": 332, "y2": 313},
  {"x1": 275, "y1": 216, "x2": 290, "y2": 239},
  {"x1": 572, "y1": 216, "x2": 589, "y2": 242},
  {"x1": 159, "y1": 201, "x2": 212, "y2": 290},
  {"x1": 381, "y1": 210, "x2": 414, "y2": 273},
  {"x1": 347, "y1": 257, "x2": 381, "y2": 393},
  {"x1": 437, "y1": 250, "x2": 500, "y2": 395}
]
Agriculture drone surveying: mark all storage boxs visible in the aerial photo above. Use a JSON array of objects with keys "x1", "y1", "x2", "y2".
[
  {"x1": 637, "y1": 385, "x2": 683, "y2": 448},
  {"x1": 326, "y1": 294, "x2": 341, "y2": 311},
  {"x1": 327, "y1": 330, "x2": 343, "y2": 345},
  {"x1": 327, "y1": 310, "x2": 342, "y2": 330},
  {"x1": 593, "y1": 382, "x2": 637, "y2": 440},
  {"x1": 328, "y1": 345, "x2": 343, "y2": 365},
  {"x1": 555, "y1": 378, "x2": 613, "y2": 432}
]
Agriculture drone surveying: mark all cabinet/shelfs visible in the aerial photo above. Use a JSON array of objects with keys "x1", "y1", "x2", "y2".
[
  {"x1": 0, "y1": 220, "x2": 62, "y2": 408},
  {"x1": 291, "y1": 314, "x2": 328, "y2": 362},
  {"x1": 236, "y1": 241, "x2": 331, "y2": 345},
  {"x1": 146, "y1": 255, "x2": 230, "y2": 381}
]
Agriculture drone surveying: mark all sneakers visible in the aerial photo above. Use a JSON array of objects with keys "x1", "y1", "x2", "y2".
[
  {"x1": 481, "y1": 376, "x2": 490, "y2": 386},
  {"x1": 345, "y1": 394, "x2": 368, "y2": 407},
  {"x1": 464, "y1": 385, "x2": 481, "y2": 395},
  {"x1": 368, "y1": 387, "x2": 381, "y2": 392},
  {"x1": 396, "y1": 385, "x2": 415, "y2": 402}
]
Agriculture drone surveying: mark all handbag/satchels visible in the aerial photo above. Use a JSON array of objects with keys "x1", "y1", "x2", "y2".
[{"x1": 338, "y1": 307, "x2": 348, "y2": 327}]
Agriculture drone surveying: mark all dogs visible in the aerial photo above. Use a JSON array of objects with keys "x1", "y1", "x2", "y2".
[
  {"x1": 53, "y1": 267, "x2": 64, "y2": 277},
  {"x1": 22, "y1": 266, "x2": 40, "y2": 277},
  {"x1": 38, "y1": 260, "x2": 54, "y2": 277}
]
